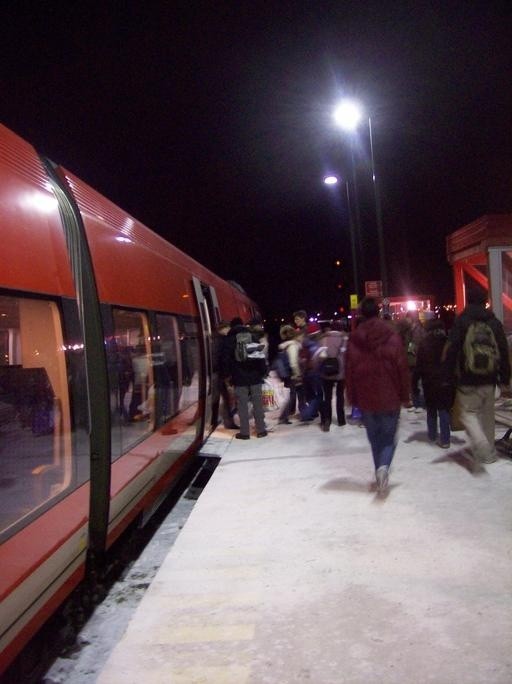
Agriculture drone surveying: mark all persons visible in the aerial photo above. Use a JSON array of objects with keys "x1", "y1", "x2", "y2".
[
  {"x1": 345, "y1": 298, "x2": 411, "y2": 498},
  {"x1": 212, "y1": 318, "x2": 269, "y2": 429},
  {"x1": 440, "y1": 285, "x2": 511, "y2": 463},
  {"x1": 278, "y1": 310, "x2": 348, "y2": 432},
  {"x1": 218, "y1": 317, "x2": 267, "y2": 440},
  {"x1": 384, "y1": 310, "x2": 455, "y2": 449}
]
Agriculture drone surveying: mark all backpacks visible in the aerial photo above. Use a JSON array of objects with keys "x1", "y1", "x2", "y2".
[
  {"x1": 462, "y1": 320, "x2": 501, "y2": 376},
  {"x1": 227, "y1": 331, "x2": 266, "y2": 386}
]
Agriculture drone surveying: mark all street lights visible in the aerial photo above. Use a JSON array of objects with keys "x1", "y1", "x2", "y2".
[
  {"x1": 330, "y1": 95, "x2": 390, "y2": 299},
  {"x1": 322, "y1": 171, "x2": 359, "y2": 301}
]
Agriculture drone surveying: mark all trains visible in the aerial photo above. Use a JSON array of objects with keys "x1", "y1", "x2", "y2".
[{"x1": 0, "y1": 121, "x2": 267, "y2": 683}]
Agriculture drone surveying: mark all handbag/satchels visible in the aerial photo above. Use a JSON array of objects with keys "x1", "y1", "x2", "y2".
[
  {"x1": 277, "y1": 351, "x2": 292, "y2": 379},
  {"x1": 321, "y1": 358, "x2": 338, "y2": 375}
]
